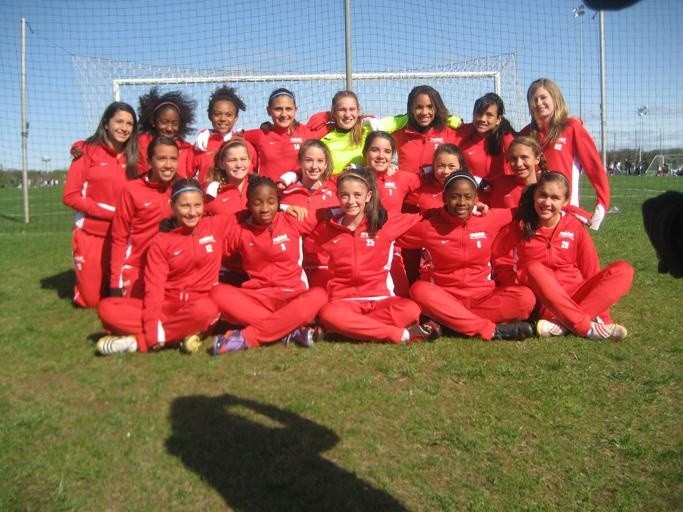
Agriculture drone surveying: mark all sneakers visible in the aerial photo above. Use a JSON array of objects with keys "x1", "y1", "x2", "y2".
[
  {"x1": 536, "y1": 319, "x2": 563, "y2": 336},
  {"x1": 284, "y1": 326, "x2": 313, "y2": 347},
  {"x1": 97, "y1": 335, "x2": 138, "y2": 355},
  {"x1": 181, "y1": 336, "x2": 200, "y2": 353},
  {"x1": 589, "y1": 322, "x2": 627, "y2": 342},
  {"x1": 408, "y1": 322, "x2": 441, "y2": 342},
  {"x1": 214, "y1": 330, "x2": 244, "y2": 355},
  {"x1": 495, "y1": 322, "x2": 532, "y2": 340}
]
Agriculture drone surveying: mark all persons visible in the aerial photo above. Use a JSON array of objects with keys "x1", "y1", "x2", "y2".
[
  {"x1": 158, "y1": 175, "x2": 344, "y2": 355},
  {"x1": 108, "y1": 86, "x2": 593, "y2": 298},
  {"x1": 607, "y1": 158, "x2": 683, "y2": 177},
  {"x1": 301, "y1": 168, "x2": 488, "y2": 344},
  {"x1": 395, "y1": 170, "x2": 537, "y2": 340},
  {"x1": 520, "y1": 78, "x2": 611, "y2": 232},
  {"x1": 95, "y1": 178, "x2": 307, "y2": 357},
  {"x1": 62, "y1": 101, "x2": 147, "y2": 310},
  {"x1": 513, "y1": 171, "x2": 633, "y2": 340},
  {"x1": 0, "y1": 177, "x2": 66, "y2": 189}
]
